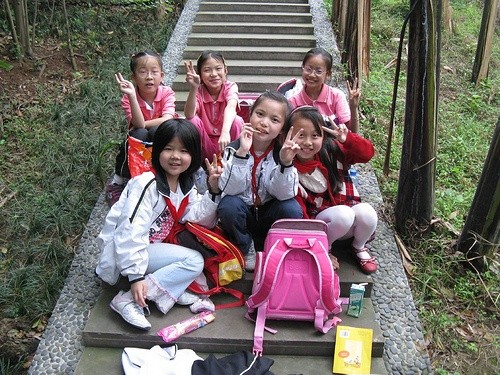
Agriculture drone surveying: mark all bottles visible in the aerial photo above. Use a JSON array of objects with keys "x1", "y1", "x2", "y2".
[{"x1": 349, "y1": 169, "x2": 358, "y2": 189}]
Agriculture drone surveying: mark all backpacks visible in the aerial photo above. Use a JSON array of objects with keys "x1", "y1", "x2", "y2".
[
  {"x1": 246, "y1": 217, "x2": 342, "y2": 333},
  {"x1": 183, "y1": 220, "x2": 247, "y2": 296},
  {"x1": 238, "y1": 95, "x2": 262, "y2": 124}
]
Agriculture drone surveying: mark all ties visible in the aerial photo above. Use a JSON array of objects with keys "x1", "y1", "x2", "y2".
[
  {"x1": 292, "y1": 153, "x2": 338, "y2": 206},
  {"x1": 249, "y1": 142, "x2": 275, "y2": 221},
  {"x1": 162, "y1": 194, "x2": 189, "y2": 245}
]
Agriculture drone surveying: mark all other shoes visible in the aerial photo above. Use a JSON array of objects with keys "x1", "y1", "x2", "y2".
[
  {"x1": 106, "y1": 183, "x2": 124, "y2": 207},
  {"x1": 327, "y1": 249, "x2": 338, "y2": 271},
  {"x1": 352, "y1": 245, "x2": 377, "y2": 274}
]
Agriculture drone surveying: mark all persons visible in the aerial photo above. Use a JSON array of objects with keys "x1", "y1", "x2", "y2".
[
  {"x1": 273, "y1": 47, "x2": 361, "y2": 145},
  {"x1": 286, "y1": 105, "x2": 378, "y2": 274},
  {"x1": 105, "y1": 52, "x2": 184, "y2": 209},
  {"x1": 96, "y1": 119, "x2": 225, "y2": 329},
  {"x1": 215, "y1": 90, "x2": 303, "y2": 274},
  {"x1": 183, "y1": 50, "x2": 247, "y2": 167}
]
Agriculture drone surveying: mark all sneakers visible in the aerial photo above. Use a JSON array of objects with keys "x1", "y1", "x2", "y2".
[
  {"x1": 109, "y1": 290, "x2": 152, "y2": 330},
  {"x1": 188, "y1": 295, "x2": 216, "y2": 314},
  {"x1": 243, "y1": 237, "x2": 257, "y2": 270},
  {"x1": 178, "y1": 291, "x2": 198, "y2": 306}
]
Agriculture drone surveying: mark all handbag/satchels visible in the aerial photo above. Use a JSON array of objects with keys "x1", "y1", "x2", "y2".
[{"x1": 126, "y1": 135, "x2": 156, "y2": 178}]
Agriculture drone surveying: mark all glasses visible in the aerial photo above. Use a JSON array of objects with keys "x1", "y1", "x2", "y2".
[{"x1": 302, "y1": 64, "x2": 328, "y2": 76}]
